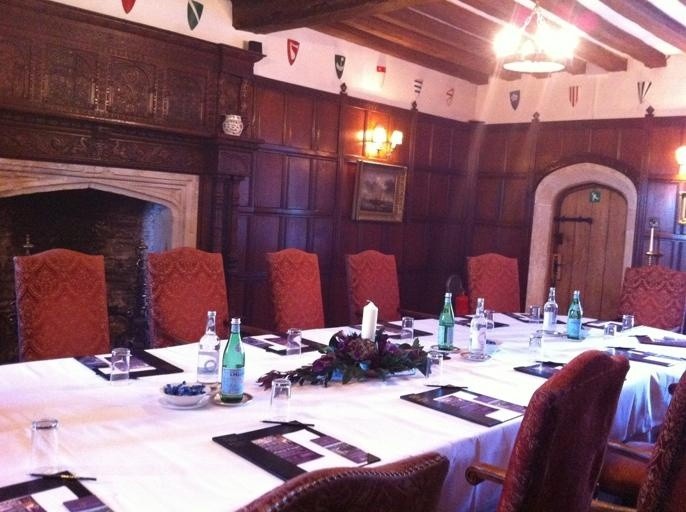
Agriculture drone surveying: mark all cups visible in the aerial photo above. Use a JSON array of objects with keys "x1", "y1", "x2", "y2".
[
  {"x1": 283, "y1": 329, "x2": 305, "y2": 366},
  {"x1": 270, "y1": 377, "x2": 292, "y2": 425},
  {"x1": 29, "y1": 417, "x2": 66, "y2": 479},
  {"x1": 526, "y1": 306, "x2": 543, "y2": 365},
  {"x1": 603, "y1": 314, "x2": 635, "y2": 344},
  {"x1": 424, "y1": 351, "x2": 445, "y2": 392},
  {"x1": 110, "y1": 347, "x2": 132, "y2": 389},
  {"x1": 484, "y1": 308, "x2": 496, "y2": 334},
  {"x1": 401, "y1": 316, "x2": 415, "y2": 345}
]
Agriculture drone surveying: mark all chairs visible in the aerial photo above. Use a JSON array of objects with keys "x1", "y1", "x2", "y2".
[
  {"x1": 346, "y1": 248, "x2": 404, "y2": 321},
  {"x1": 231, "y1": 453, "x2": 449, "y2": 511},
  {"x1": 592, "y1": 372, "x2": 684, "y2": 511},
  {"x1": 9, "y1": 246, "x2": 120, "y2": 360},
  {"x1": 266, "y1": 245, "x2": 324, "y2": 331},
  {"x1": 466, "y1": 251, "x2": 521, "y2": 313},
  {"x1": 464, "y1": 347, "x2": 630, "y2": 511},
  {"x1": 619, "y1": 258, "x2": 684, "y2": 333},
  {"x1": 147, "y1": 244, "x2": 230, "y2": 351}
]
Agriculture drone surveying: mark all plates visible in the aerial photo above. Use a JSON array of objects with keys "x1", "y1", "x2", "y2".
[{"x1": 160, "y1": 395, "x2": 209, "y2": 410}]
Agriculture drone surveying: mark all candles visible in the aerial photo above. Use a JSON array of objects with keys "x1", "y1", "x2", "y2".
[{"x1": 645, "y1": 223, "x2": 658, "y2": 270}]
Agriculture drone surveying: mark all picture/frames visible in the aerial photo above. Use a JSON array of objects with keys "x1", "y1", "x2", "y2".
[{"x1": 351, "y1": 160, "x2": 409, "y2": 224}]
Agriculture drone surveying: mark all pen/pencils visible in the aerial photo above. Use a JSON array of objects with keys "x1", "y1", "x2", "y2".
[
  {"x1": 260, "y1": 421, "x2": 314, "y2": 426},
  {"x1": 424, "y1": 385, "x2": 468, "y2": 388},
  {"x1": 29, "y1": 473, "x2": 96, "y2": 480}
]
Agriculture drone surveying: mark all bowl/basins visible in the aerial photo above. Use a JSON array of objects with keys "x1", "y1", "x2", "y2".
[{"x1": 164, "y1": 383, "x2": 208, "y2": 405}]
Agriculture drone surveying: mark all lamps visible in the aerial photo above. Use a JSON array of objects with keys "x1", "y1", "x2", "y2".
[
  {"x1": 674, "y1": 145, "x2": 686, "y2": 225},
  {"x1": 374, "y1": 127, "x2": 403, "y2": 150},
  {"x1": 490, "y1": 3, "x2": 580, "y2": 74}
]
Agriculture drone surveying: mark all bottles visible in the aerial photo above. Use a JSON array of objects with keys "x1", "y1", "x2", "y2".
[
  {"x1": 543, "y1": 285, "x2": 583, "y2": 338},
  {"x1": 195, "y1": 310, "x2": 246, "y2": 402},
  {"x1": 465, "y1": 298, "x2": 489, "y2": 359},
  {"x1": 223, "y1": 115, "x2": 244, "y2": 137},
  {"x1": 437, "y1": 293, "x2": 454, "y2": 348}
]
